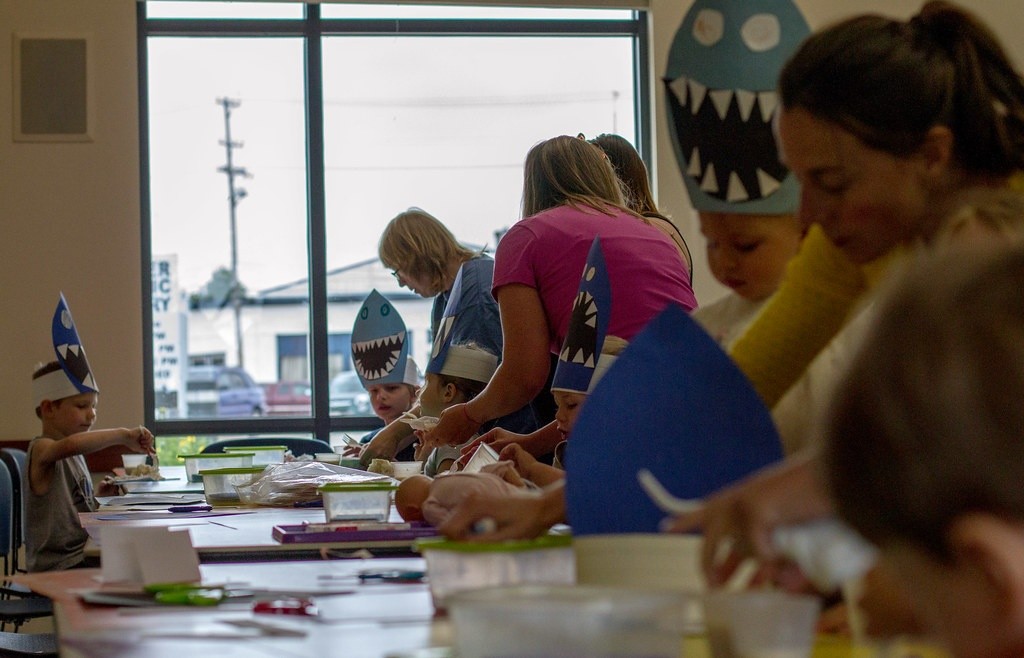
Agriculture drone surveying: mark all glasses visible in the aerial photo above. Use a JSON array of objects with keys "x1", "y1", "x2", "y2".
[{"x1": 391, "y1": 268, "x2": 401, "y2": 280}]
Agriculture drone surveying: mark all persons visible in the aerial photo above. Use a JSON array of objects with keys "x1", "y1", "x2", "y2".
[
  {"x1": 343, "y1": 0, "x2": 1024, "y2": 658},
  {"x1": 24, "y1": 360, "x2": 158, "y2": 573},
  {"x1": 395, "y1": 473, "x2": 520, "y2": 527}
]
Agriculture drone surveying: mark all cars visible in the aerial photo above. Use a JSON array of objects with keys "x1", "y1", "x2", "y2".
[
  {"x1": 187, "y1": 364, "x2": 267, "y2": 418},
  {"x1": 330, "y1": 370, "x2": 376, "y2": 416},
  {"x1": 260, "y1": 382, "x2": 312, "y2": 417}
]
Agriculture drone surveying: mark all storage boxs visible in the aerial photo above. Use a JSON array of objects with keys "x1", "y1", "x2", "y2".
[
  {"x1": 178, "y1": 453, "x2": 256, "y2": 482},
  {"x1": 223, "y1": 446, "x2": 288, "y2": 465},
  {"x1": 198, "y1": 468, "x2": 266, "y2": 504},
  {"x1": 446, "y1": 585, "x2": 682, "y2": 658},
  {"x1": 318, "y1": 482, "x2": 399, "y2": 523},
  {"x1": 412, "y1": 530, "x2": 574, "y2": 611}
]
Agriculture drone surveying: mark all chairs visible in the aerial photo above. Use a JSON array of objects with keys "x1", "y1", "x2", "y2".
[{"x1": 0, "y1": 448, "x2": 58, "y2": 658}]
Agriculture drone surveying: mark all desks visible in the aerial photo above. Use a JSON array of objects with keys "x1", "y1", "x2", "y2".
[{"x1": 54, "y1": 459, "x2": 577, "y2": 658}]
